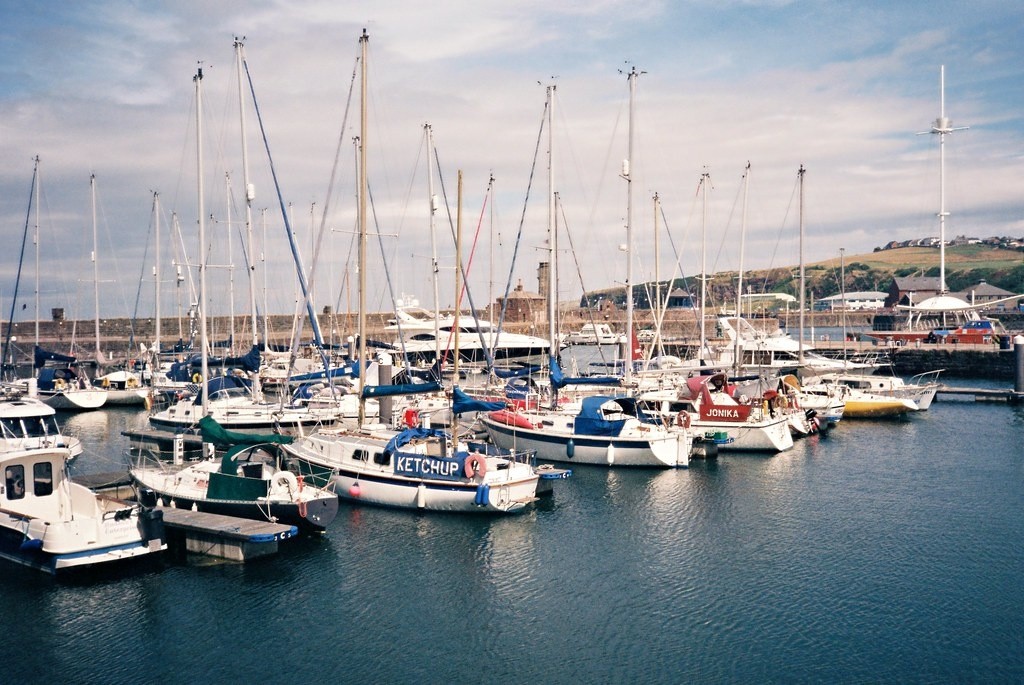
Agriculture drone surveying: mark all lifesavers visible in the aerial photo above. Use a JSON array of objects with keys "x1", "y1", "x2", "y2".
[
  {"x1": 192, "y1": 373, "x2": 202, "y2": 383},
  {"x1": 272, "y1": 472, "x2": 297, "y2": 496},
  {"x1": 463, "y1": 454, "x2": 486, "y2": 479},
  {"x1": 677, "y1": 410, "x2": 691, "y2": 429},
  {"x1": 55, "y1": 378, "x2": 64, "y2": 390}
]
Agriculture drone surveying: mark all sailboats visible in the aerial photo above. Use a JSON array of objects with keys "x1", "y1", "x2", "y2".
[{"x1": 0, "y1": 25, "x2": 1020, "y2": 574}]
